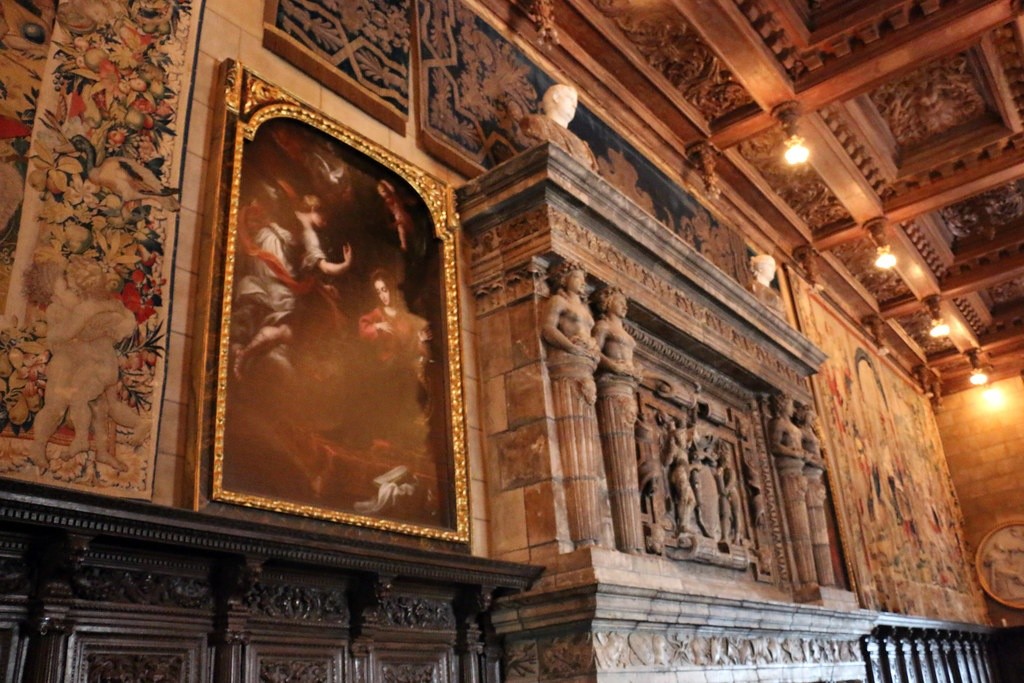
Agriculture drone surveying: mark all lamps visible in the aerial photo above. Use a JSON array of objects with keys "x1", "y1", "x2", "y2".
[
  {"x1": 771, "y1": 100, "x2": 811, "y2": 168},
  {"x1": 962, "y1": 348, "x2": 989, "y2": 386},
  {"x1": 863, "y1": 214, "x2": 898, "y2": 270},
  {"x1": 922, "y1": 292, "x2": 951, "y2": 339}
]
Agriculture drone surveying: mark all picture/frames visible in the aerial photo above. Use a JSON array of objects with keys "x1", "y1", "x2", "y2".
[{"x1": 186, "y1": 56, "x2": 472, "y2": 558}]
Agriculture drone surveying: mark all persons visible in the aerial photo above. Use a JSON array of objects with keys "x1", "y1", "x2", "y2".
[
  {"x1": 543, "y1": 251, "x2": 832, "y2": 584},
  {"x1": 519, "y1": 84, "x2": 599, "y2": 171}
]
